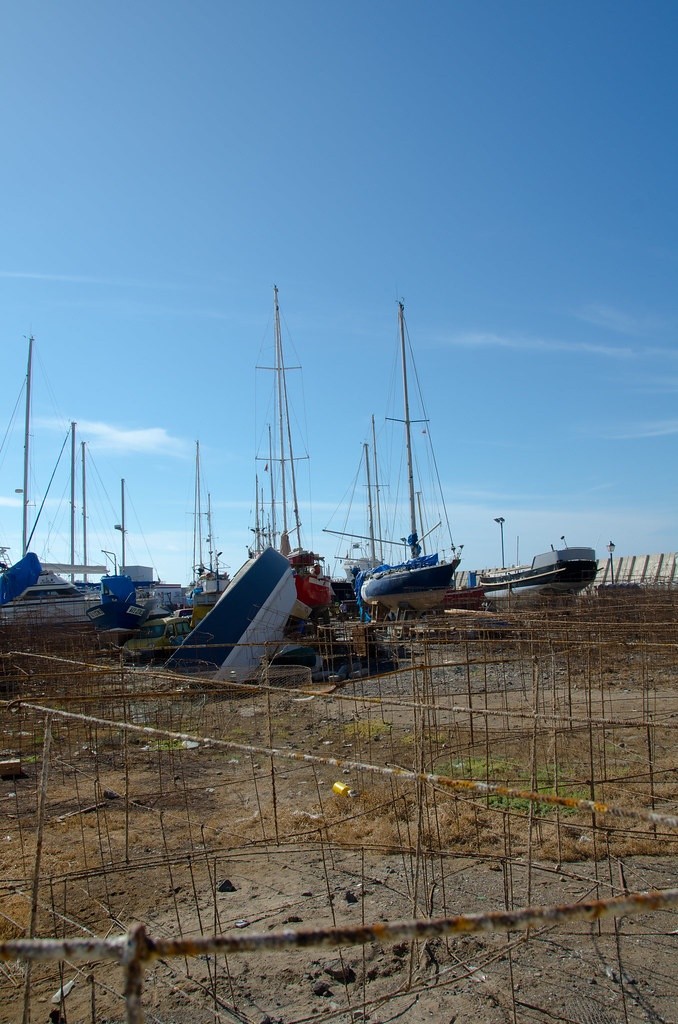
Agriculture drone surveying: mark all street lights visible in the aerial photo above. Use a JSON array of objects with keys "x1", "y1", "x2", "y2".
[
  {"x1": 607, "y1": 541, "x2": 615, "y2": 585},
  {"x1": 494, "y1": 517, "x2": 505, "y2": 569}
]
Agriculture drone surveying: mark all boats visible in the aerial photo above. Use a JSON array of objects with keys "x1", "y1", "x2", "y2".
[
  {"x1": 479, "y1": 547, "x2": 596, "y2": 607},
  {"x1": 166, "y1": 545, "x2": 298, "y2": 684},
  {"x1": 121, "y1": 616, "x2": 192, "y2": 659}
]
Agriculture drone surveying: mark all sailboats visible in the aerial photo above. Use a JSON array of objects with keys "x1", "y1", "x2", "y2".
[
  {"x1": 321, "y1": 297, "x2": 464, "y2": 613},
  {"x1": 0, "y1": 281, "x2": 334, "y2": 633}
]
